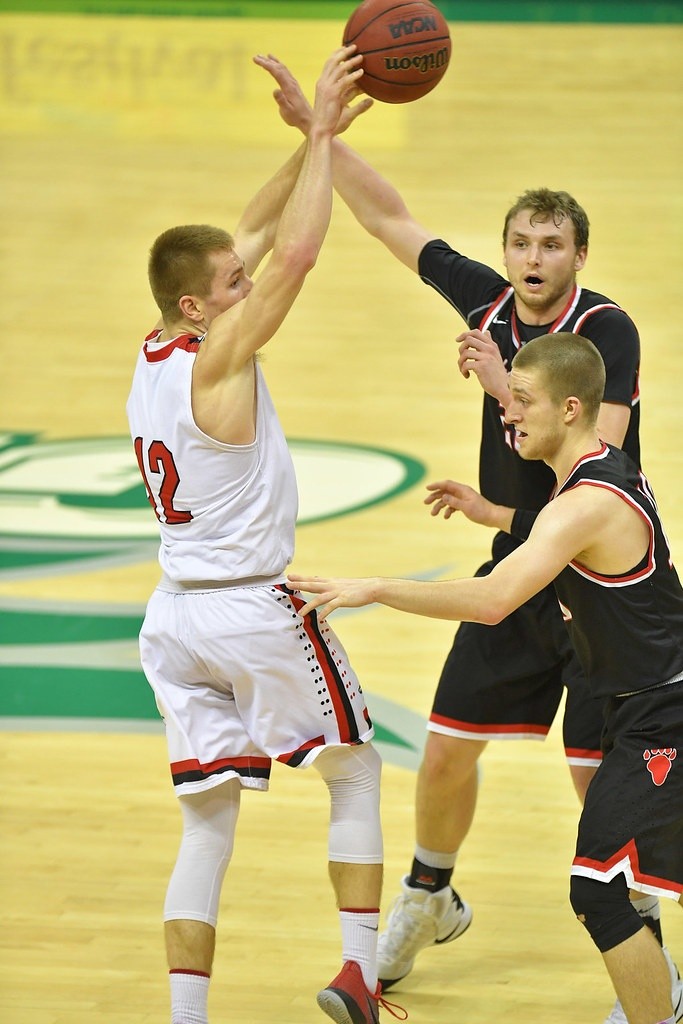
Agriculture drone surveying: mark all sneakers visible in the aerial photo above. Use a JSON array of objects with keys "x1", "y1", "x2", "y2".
[
  {"x1": 606, "y1": 951, "x2": 683, "y2": 1024},
  {"x1": 316, "y1": 959, "x2": 408, "y2": 1024},
  {"x1": 375, "y1": 876, "x2": 474, "y2": 990}
]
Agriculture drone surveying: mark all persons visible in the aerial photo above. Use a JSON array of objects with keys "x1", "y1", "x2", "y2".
[
  {"x1": 252, "y1": 52, "x2": 683, "y2": 1024},
  {"x1": 128, "y1": 46, "x2": 408, "y2": 1024},
  {"x1": 287, "y1": 333, "x2": 682, "y2": 1024}
]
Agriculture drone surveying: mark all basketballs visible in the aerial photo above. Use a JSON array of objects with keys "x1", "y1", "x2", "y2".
[{"x1": 341, "y1": 0, "x2": 453, "y2": 104}]
